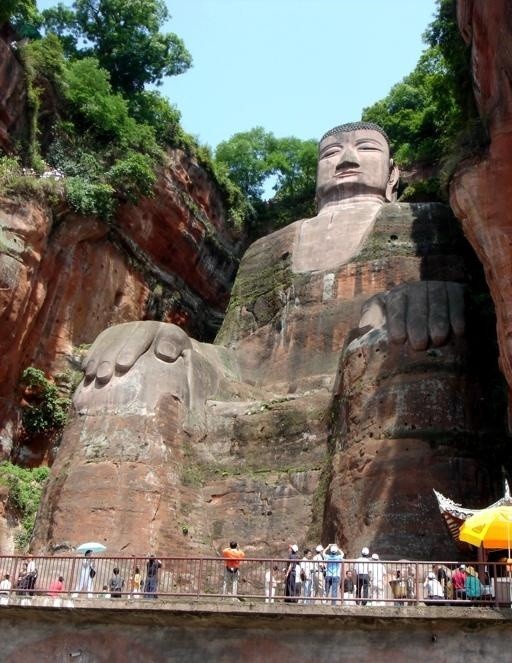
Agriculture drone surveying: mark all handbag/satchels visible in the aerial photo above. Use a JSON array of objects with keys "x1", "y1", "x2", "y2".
[
  {"x1": 90, "y1": 569, "x2": 95, "y2": 577},
  {"x1": 228, "y1": 567, "x2": 237, "y2": 573},
  {"x1": 300, "y1": 568, "x2": 305, "y2": 581}
]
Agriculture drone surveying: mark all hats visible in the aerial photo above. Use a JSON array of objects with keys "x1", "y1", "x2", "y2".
[
  {"x1": 316, "y1": 545, "x2": 324, "y2": 552},
  {"x1": 289, "y1": 545, "x2": 298, "y2": 552},
  {"x1": 460, "y1": 565, "x2": 475, "y2": 576},
  {"x1": 330, "y1": 546, "x2": 337, "y2": 553},
  {"x1": 362, "y1": 548, "x2": 369, "y2": 555},
  {"x1": 372, "y1": 554, "x2": 379, "y2": 559}
]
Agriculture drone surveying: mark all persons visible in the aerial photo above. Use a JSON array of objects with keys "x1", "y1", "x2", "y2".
[{"x1": 29, "y1": 120, "x2": 481, "y2": 563}]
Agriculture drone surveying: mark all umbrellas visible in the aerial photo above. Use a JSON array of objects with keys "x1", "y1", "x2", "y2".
[{"x1": 459, "y1": 506, "x2": 512, "y2": 555}]
